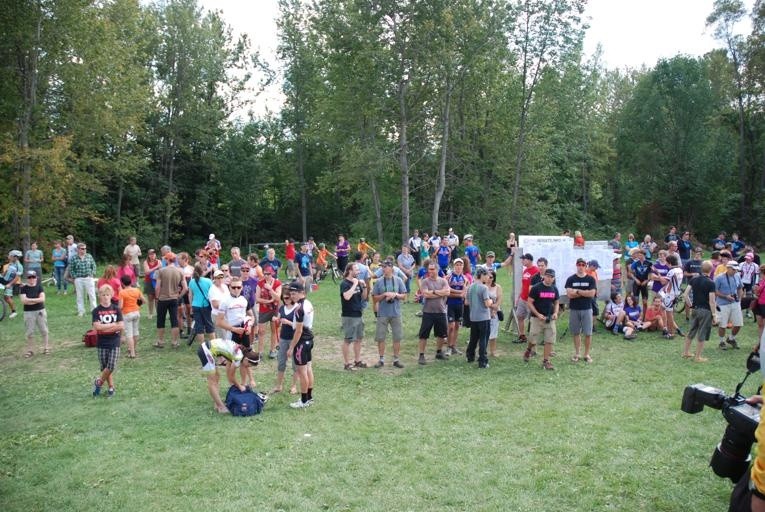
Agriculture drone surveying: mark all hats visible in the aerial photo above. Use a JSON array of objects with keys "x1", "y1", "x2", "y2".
[
  {"x1": 241, "y1": 264, "x2": 250, "y2": 269},
  {"x1": 148, "y1": 248, "x2": 155, "y2": 253},
  {"x1": 288, "y1": 282, "x2": 304, "y2": 291},
  {"x1": 163, "y1": 252, "x2": 177, "y2": 262},
  {"x1": 744, "y1": 252, "x2": 754, "y2": 260},
  {"x1": 379, "y1": 259, "x2": 394, "y2": 267},
  {"x1": 544, "y1": 269, "x2": 555, "y2": 278},
  {"x1": 576, "y1": 258, "x2": 585, "y2": 264},
  {"x1": 26, "y1": 270, "x2": 37, "y2": 276},
  {"x1": 209, "y1": 234, "x2": 215, "y2": 239},
  {"x1": 520, "y1": 253, "x2": 533, "y2": 261},
  {"x1": 589, "y1": 259, "x2": 601, "y2": 268},
  {"x1": 453, "y1": 257, "x2": 465, "y2": 264},
  {"x1": 66, "y1": 234, "x2": 74, "y2": 240},
  {"x1": 213, "y1": 270, "x2": 223, "y2": 276},
  {"x1": 485, "y1": 251, "x2": 495, "y2": 257},
  {"x1": 726, "y1": 260, "x2": 740, "y2": 270}
]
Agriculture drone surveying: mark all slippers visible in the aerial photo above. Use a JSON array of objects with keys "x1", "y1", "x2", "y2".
[
  {"x1": 584, "y1": 356, "x2": 593, "y2": 363},
  {"x1": 571, "y1": 355, "x2": 580, "y2": 362}
]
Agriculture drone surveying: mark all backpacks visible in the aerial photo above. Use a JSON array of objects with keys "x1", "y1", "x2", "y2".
[{"x1": 0, "y1": 266, "x2": 17, "y2": 285}]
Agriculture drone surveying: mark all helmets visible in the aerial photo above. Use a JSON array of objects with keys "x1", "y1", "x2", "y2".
[
  {"x1": 8, "y1": 250, "x2": 23, "y2": 257},
  {"x1": 463, "y1": 234, "x2": 473, "y2": 241},
  {"x1": 360, "y1": 238, "x2": 366, "y2": 243},
  {"x1": 308, "y1": 236, "x2": 314, "y2": 240},
  {"x1": 318, "y1": 242, "x2": 325, "y2": 248}
]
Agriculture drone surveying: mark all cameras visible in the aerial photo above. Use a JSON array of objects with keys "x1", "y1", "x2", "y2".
[
  {"x1": 545, "y1": 316, "x2": 551, "y2": 323},
  {"x1": 387, "y1": 299, "x2": 394, "y2": 304},
  {"x1": 681, "y1": 384, "x2": 760, "y2": 483},
  {"x1": 731, "y1": 293, "x2": 739, "y2": 301}
]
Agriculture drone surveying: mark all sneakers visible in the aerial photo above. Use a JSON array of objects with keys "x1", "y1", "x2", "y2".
[
  {"x1": 447, "y1": 346, "x2": 462, "y2": 356},
  {"x1": 543, "y1": 361, "x2": 554, "y2": 369},
  {"x1": 9, "y1": 312, "x2": 18, "y2": 319},
  {"x1": 418, "y1": 353, "x2": 426, "y2": 365},
  {"x1": 345, "y1": 361, "x2": 366, "y2": 371},
  {"x1": 726, "y1": 337, "x2": 740, "y2": 349},
  {"x1": 436, "y1": 352, "x2": 448, "y2": 361},
  {"x1": 93, "y1": 378, "x2": 104, "y2": 397},
  {"x1": 624, "y1": 335, "x2": 637, "y2": 339},
  {"x1": 108, "y1": 386, "x2": 115, "y2": 396},
  {"x1": 289, "y1": 398, "x2": 313, "y2": 409},
  {"x1": 719, "y1": 341, "x2": 726, "y2": 350},
  {"x1": 522, "y1": 348, "x2": 536, "y2": 363}
]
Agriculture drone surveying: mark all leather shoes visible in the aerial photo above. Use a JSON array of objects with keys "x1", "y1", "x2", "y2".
[
  {"x1": 374, "y1": 361, "x2": 384, "y2": 368},
  {"x1": 392, "y1": 361, "x2": 404, "y2": 368},
  {"x1": 512, "y1": 338, "x2": 526, "y2": 343}
]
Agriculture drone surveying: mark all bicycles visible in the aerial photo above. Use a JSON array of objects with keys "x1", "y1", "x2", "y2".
[
  {"x1": 676, "y1": 286, "x2": 686, "y2": 314},
  {"x1": 314, "y1": 251, "x2": 344, "y2": 289},
  {"x1": 364, "y1": 252, "x2": 377, "y2": 268},
  {"x1": 185, "y1": 315, "x2": 198, "y2": 345},
  {"x1": 0, "y1": 298, "x2": 6, "y2": 321}
]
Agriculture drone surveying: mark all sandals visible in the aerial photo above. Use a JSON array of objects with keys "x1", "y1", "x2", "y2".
[
  {"x1": 24, "y1": 351, "x2": 34, "y2": 358},
  {"x1": 152, "y1": 343, "x2": 165, "y2": 348},
  {"x1": 172, "y1": 343, "x2": 180, "y2": 349},
  {"x1": 43, "y1": 349, "x2": 51, "y2": 355}
]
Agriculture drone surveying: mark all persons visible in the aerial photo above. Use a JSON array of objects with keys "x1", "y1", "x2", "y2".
[
  {"x1": 743, "y1": 380, "x2": 764, "y2": 512},
  {"x1": 92, "y1": 228, "x2": 518, "y2": 414},
  {"x1": 0, "y1": 236, "x2": 96, "y2": 359},
  {"x1": 512, "y1": 227, "x2": 765, "y2": 371}
]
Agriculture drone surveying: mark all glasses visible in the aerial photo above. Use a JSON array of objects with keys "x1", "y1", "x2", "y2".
[
  {"x1": 429, "y1": 268, "x2": 436, "y2": 271},
  {"x1": 264, "y1": 273, "x2": 271, "y2": 276},
  {"x1": 283, "y1": 296, "x2": 290, "y2": 299},
  {"x1": 577, "y1": 264, "x2": 585, "y2": 266},
  {"x1": 230, "y1": 285, "x2": 243, "y2": 289},
  {"x1": 241, "y1": 269, "x2": 249, "y2": 272},
  {"x1": 222, "y1": 269, "x2": 228, "y2": 272},
  {"x1": 80, "y1": 247, "x2": 86, "y2": 250},
  {"x1": 217, "y1": 276, "x2": 223, "y2": 279},
  {"x1": 29, "y1": 275, "x2": 36, "y2": 279}
]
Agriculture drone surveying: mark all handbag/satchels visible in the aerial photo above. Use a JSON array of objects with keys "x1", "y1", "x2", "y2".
[
  {"x1": 225, "y1": 383, "x2": 264, "y2": 416},
  {"x1": 496, "y1": 310, "x2": 504, "y2": 321},
  {"x1": 656, "y1": 273, "x2": 682, "y2": 308}
]
